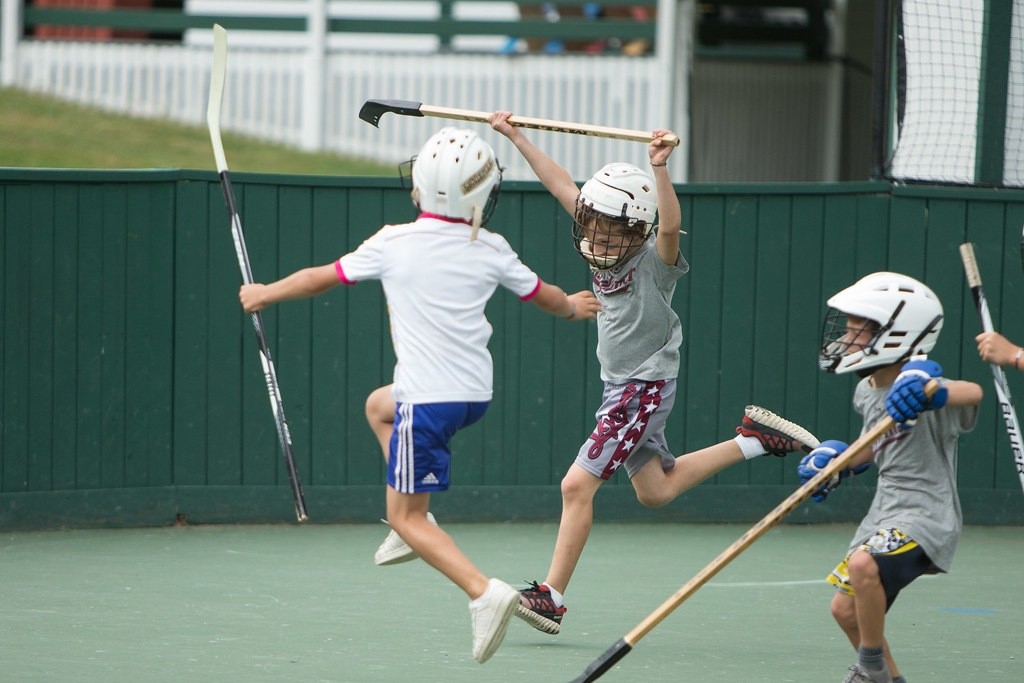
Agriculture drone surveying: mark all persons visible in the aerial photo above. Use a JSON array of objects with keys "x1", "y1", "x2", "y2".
[
  {"x1": 237, "y1": 126, "x2": 606, "y2": 662},
  {"x1": 485, "y1": 110, "x2": 825, "y2": 636},
  {"x1": 974, "y1": 331, "x2": 1024, "y2": 371},
  {"x1": 800, "y1": 271, "x2": 986, "y2": 683}
]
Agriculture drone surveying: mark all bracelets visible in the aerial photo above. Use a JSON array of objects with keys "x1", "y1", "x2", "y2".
[
  {"x1": 650, "y1": 161, "x2": 666, "y2": 166},
  {"x1": 565, "y1": 301, "x2": 576, "y2": 320},
  {"x1": 1015, "y1": 348, "x2": 1024, "y2": 370}
]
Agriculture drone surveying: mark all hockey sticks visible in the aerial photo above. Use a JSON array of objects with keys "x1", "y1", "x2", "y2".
[
  {"x1": 959, "y1": 239, "x2": 1024, "y2": 490},
  {"x1": 567, "y1": 377, "x2": 943, "y2": 683},
  {"x1": 207, "y1": 21, "x2": 309, "y2": 526},
  {"x1": 357, "y1": 99, "x2": 682, "y2": 147}
]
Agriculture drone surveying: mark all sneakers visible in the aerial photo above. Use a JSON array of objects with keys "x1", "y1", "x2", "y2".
[
  {"x1": 843, "y1": 657, "x2": 892, "y2": 683},
  {"x1": 375, "y1": 511, "x2": 438, "y2": 564},
  {"x1": 468, "y1": 577, "x2": 521, "y2": 664},
  {"x1": 512, "y1": 580, "x2": 567, "y2": 635},
  {"x1": 736, "y1": 404, "x2": 821, "y2": 456}
]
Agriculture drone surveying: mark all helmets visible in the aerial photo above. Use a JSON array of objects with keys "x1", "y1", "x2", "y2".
[
  {"x1": 572, "y1": 161, "x2": 658, "y2": 269},
  {"x1": 399, "y1": 127, "x2": 503, "y2": 228},
  {"x1": 818, "y1": 271, "x2": 944, "y2": 378}
]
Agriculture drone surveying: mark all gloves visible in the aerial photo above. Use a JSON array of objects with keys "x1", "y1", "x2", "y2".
[
  {"x1": 885, "y1": 360, "x2": 948, "y2": 429},
  {"x1": 797, "y1": 440, "x2": 871, "y2": 503}
]
